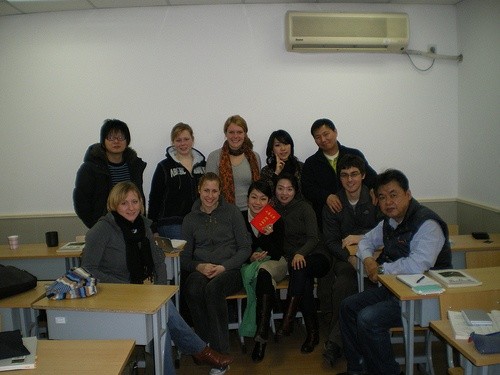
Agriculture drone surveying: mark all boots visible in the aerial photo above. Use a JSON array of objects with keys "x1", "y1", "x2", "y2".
[{"x1": 277, "y1": 295, "x2": 298, "y2": 336}]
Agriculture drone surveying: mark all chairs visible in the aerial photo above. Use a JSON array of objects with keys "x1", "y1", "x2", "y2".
[
  {"x1": 387, "y1": 327, "x2": 434, "y2": 374},
  {"x1": 227, "y1": 278, "x2": 317, "y2": 355}
]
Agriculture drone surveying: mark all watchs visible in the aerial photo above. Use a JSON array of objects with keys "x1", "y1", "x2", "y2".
[{"x1": 378, "y1": 264, "x2": 385, "y2": 276}]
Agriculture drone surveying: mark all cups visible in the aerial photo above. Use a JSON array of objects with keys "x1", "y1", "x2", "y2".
[
  {"x1": 8, "y1": 236, "x2": 19, "y2": 249},
  {"x1": 46, "y1": 232, "x2": 58, "y2": 247}
]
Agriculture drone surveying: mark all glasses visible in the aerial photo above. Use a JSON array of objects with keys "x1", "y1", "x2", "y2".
[
  {"x1": 339, "y1": 172, "x2": 361, "y2": 178},
  {"x1": 105, "y1": 136, "x2": 126, "y2": 141}
]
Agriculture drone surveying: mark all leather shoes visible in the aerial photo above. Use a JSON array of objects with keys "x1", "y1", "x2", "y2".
[
  {"x1": 301, "y1": 329, "x2": 320, "y2": 353},
  {"x1": 252, "y1": 335, "x2": 266, "y2": 362},
  {"x1": 322, "y1": 341, "x2": 341, "y2": 368},
  {"x1": 193, "y1": 344, "x2": 233, "y2": 367}
]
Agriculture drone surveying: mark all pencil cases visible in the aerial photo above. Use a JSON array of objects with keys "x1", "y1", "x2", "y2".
[
  {"x1": 468, "y1": 332, "x2": 500, "y2": 355},
  {"x1": 472, "y1": 232, "x2": 490, "y2": 240},
  {"x1": 0, "y1": 330, "x2": 31, "y2": 361}
]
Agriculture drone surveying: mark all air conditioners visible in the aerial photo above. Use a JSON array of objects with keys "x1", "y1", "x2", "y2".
[{"x1": 284, "y1": 8, "x2": 410, "y2": 55}]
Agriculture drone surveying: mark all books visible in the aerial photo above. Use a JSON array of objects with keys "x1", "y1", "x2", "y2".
[
  {"x1": 152, "y1": 236, "x2": 188, "y2": 253},
  {"x1": 396, "y1": 273, "x2": 445, "y2": 295},
  {"x1": 0, "y1": 335, "x2": 36, "y2": 371},
  {"x1": 56, "y1": 241, "x2": 86, "y2": 253},
  {"x1": 447, "y1": 308, "x2": 500, "y2": 341},
  {"x1": 429, "y1": 268, "x2": 484, "y2": 288}
]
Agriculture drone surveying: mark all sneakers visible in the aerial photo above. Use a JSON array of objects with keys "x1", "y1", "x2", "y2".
[{"x1": 210, "y1": 365, "x2": 230, "y2": 375}]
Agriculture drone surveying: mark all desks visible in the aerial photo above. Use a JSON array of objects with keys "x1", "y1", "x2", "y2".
[{"x1": 0, "y1": 225, "x2": 500, "y2": 374}]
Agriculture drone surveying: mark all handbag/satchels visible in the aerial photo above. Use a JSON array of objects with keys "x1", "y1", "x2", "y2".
[{"x1": 0, "y1": 263, "x2": 37, "y2": 300}]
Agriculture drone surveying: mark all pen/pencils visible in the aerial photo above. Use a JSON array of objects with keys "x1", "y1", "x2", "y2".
[{"x1": 417, "y1": 276, "x2": 425, "y2": 283}]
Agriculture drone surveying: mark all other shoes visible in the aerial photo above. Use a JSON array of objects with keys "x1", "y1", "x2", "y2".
[{"x1": 336, "y1": 371, "x2": 368, "y2": 375}]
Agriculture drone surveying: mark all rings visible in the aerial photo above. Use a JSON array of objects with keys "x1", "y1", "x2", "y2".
[{"x1": 268, "y1": 227, "x2": 272, "y2": 230}]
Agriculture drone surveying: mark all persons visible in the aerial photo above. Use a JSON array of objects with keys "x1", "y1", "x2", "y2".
[
  {"x1": 322, "y1": 154, "x2": 386, "y2": 367},
  {"x1": 332, "y1": 169, "x2": 453, "y2": 375},
  {"x1": 72, "y1": 118, "x2": 147, "y2": 229},
  {"x1": 206, "y1": 115, "x2": 261, "y2": 222},
  {"x1": 80, "y1": 181, "x2": 233, "y2": 375},
  {"x1": 260, "y1": 129, "x2": 305, "y2": 200},
  {"x1": 303, "y1": 118, "x2": 379, "y2": 222},
  {"x1": 238, "y1": 180, "x2": 288, "y2": 361},
  {"x1": 149, "y1": 122, "x2": 208, "y2": 240},
  {"x1": 268, "y1": 174, "x2": 320, "y2": 353},
  {"x1": 179, "y1": 172, "x2": 253, "y2": 375}
]
